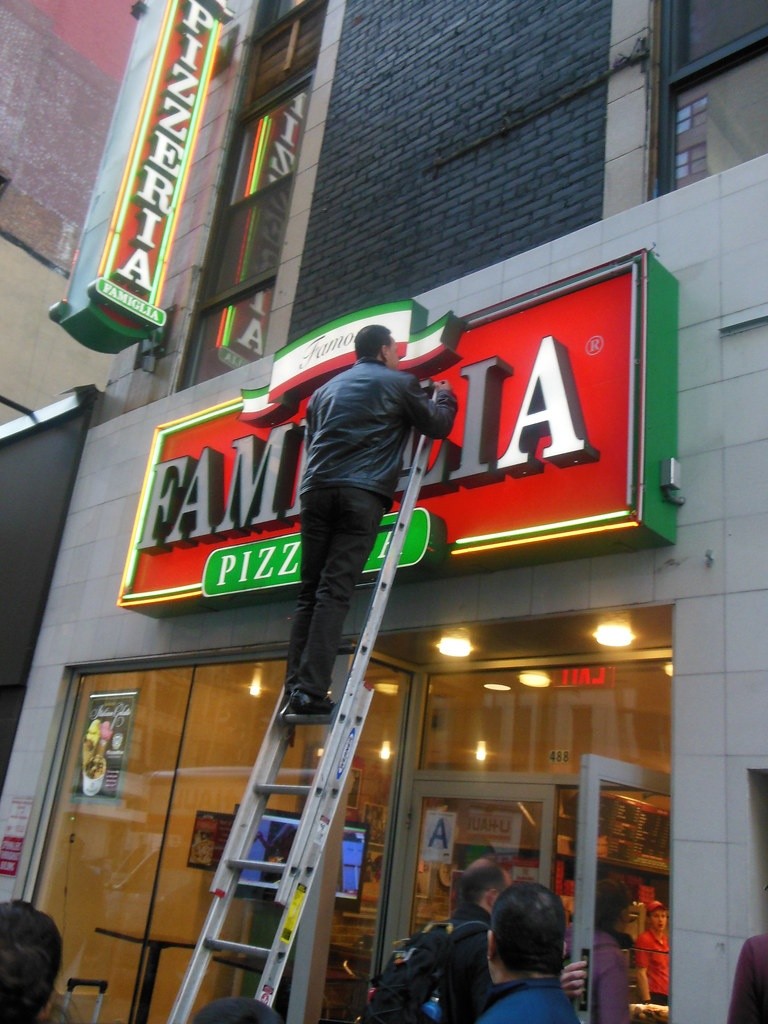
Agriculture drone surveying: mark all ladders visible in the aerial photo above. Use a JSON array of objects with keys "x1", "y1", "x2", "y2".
[{"x1": 170, "y1": 382, "x2": 437, "y2": 1014}]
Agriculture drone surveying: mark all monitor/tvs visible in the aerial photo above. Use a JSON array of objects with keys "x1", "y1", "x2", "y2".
[{"x1": 232, "y1": 804, "x2": 371, "y2": 912}]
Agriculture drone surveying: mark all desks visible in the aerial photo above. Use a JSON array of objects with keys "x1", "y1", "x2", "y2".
[{"x1": 95, "y1": 928, "x2": 197, "y2": 1024}]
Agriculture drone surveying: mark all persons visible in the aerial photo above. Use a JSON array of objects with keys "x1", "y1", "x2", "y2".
[
  {"x1": 633, "y1": 901, "x2": 671, "y2": 1006},
  {"x1": 440, "y1": 858, "x2": 588, "y2": 1024},
  {"x1": 727, "y1": 934, "x2": 768, "y2": 1024},
  {"x1": 283, "y1": 326, "x2": 457, "y2": 714},
  {"x1": 194, "y1": 996, "x2": 284, "y2": 1024},
  {"x1": 563, "y1": 877, "x2": 636, "y2": 1024},
  {"x1": 0, "y1": 899, "x2": 64, "y2": 1024}
]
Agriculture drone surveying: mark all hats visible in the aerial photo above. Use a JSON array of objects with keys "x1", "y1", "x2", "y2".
[{"x1": 646, "y1": 901, "x2": 666, "y2": 911}]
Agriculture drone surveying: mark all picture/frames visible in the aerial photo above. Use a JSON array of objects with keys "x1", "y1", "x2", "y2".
[{"x1": 346, "y1": 765, "x2": 453, "y2": 900}]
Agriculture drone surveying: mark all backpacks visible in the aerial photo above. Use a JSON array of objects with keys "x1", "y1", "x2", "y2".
[{"x1": 356, "y1": 919, "x2": 490, "y2": 1024}]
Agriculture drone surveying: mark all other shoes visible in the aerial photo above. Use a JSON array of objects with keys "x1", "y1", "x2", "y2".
[{"x1": 289, "y1": 689, "x2": 336, "y2": 713}]
